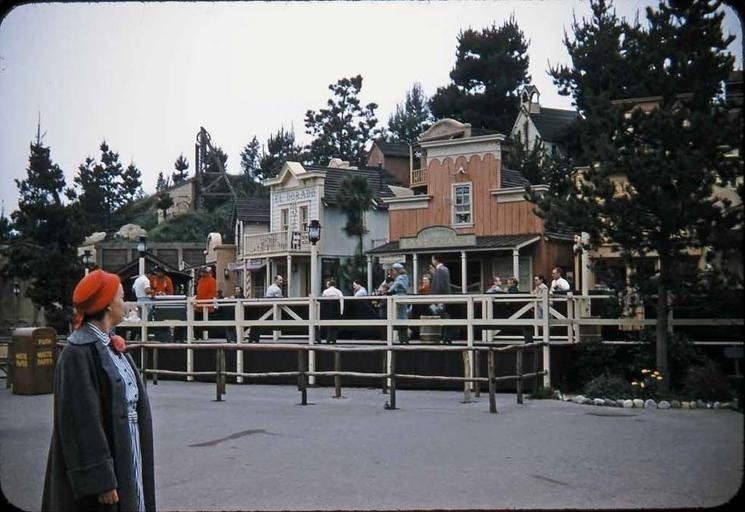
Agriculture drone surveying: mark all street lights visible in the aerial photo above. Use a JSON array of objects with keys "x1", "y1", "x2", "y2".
[
  {"x1": 136, "y1": 234, "x2": 151, "y2": 301},
  {"x1": 308, "y1": 218, "x2": 322, "y2": 297},
  {"x1": 13, "y1": 282, "x2": 21, "y2": 322},
  {"x1": 83, "y1": 250, "x2": 94, "y2": 276}
]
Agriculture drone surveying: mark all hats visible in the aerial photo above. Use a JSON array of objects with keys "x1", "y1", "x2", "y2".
[
  {"x1": 392, "y1": 263, "x2": 404, "y2": 269},
  {"x1": 72, "y1": 269, "x2": 121, "y2": 316}
]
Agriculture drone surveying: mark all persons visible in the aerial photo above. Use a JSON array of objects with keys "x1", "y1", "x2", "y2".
[
  {"x1": 353, "y1": 279, "x2": 368, "y2": 296},
  {"x1": 195, "y1": 266, "x2": 245, "y2": 343},
  {"x1": 321, "y1": 279, "x2": 344, "y2": 343},
  {"x1": 378, "y1": 262, "x2": 410, "y2": 344},
  {"x1": 485, "y1": 275, "x2": 518, "y2": 293},
  {"x1": 266, "y1": 274, "x2": 284, "y2": 298},
  {"x1": 419, "y1": 254, "x2": 452, "y2": 344},
  {"x1": 567, "y1": 276, "x2": 574, "y2": 292},
  {"x1": 40, "y1": 267, "x2": 156, "y2": 511},
  {"x1": 530, "y1": 267, "x2": 570, "y2": 336},
  {"x1": 134, "y1": 267, "x2": 173, "y2": 344}
]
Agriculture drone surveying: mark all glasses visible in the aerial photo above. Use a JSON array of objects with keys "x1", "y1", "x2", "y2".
[{"x1": 534, "y1": 279, "x2": 538, "y2": 282}]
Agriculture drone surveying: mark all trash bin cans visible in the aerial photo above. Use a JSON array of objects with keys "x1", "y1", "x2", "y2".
[
  {"x1": 11, "y1": 326, "x2": 57, "y2": 395},
  {"x1": 420, "y1": 316, "x2": 441, "y2": 341}
]
